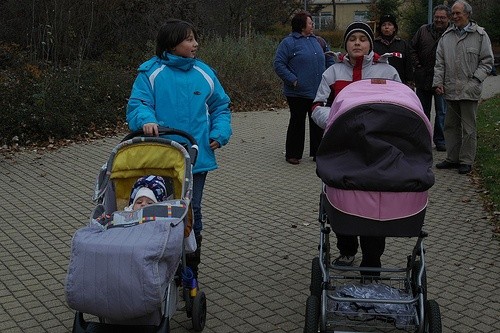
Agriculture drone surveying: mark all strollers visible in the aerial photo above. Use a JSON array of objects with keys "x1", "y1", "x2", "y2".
[
  {"x1": 303, "y1": 77, "x2": 443, "y2": 333},
  {"x1": 64, "y1": 126, "x2": 207, "y2": 333}
]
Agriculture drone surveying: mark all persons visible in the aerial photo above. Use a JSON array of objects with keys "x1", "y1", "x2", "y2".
[
  {"x1": 129, "y1": 175, "x2": 166, "y2": 211},
  {"x1": 373, "y1": 13, "x2": 412, "y2": 90},
  {"x1": 433, "y1": 0, "x2": 494, "y2": 174},
  {"x1": 126, "y1": 19, "x2": 233, "y2": 296},
  {"x1": 311, "y1": 21, "x2": 401, "y2": 286},
  {"x1": 274, "y1": 11, "x2": 335, "y2": 165},
  {"x1": 411, "y1": 6, "x2": 451, "y2": 152}
]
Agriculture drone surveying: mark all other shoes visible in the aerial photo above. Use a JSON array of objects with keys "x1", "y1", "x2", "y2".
[
  {"x1": 360, "y1": 279, "x2": 378, "y2": 285},
  {"x1": 456, "y1": 161, "x2": 472, "y2": 174},
  {"x1": 331, "y1": 253, "x2": 355, "y2": 265},
  {"x1": 286, "y1": 157, "x2": 299, "y2": 164},
  {"x1": 435, "y1": 146, "x2": 447, "y2": 152},
  {"x1": 436, "y1": 158, "x2": 458, "y2": 169}
]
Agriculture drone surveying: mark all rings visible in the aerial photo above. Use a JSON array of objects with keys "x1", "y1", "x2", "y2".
[{"x1": 216, "y1": 145, "x2": 218, "y2": 148}]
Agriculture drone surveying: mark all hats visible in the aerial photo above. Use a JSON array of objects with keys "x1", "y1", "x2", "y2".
[
  {"x1": 344, "y1": 22, "x2": 374, "y2": 52},
  {"x1": 133, "y1": 187, "x2": 157, "y2": 206}
]
